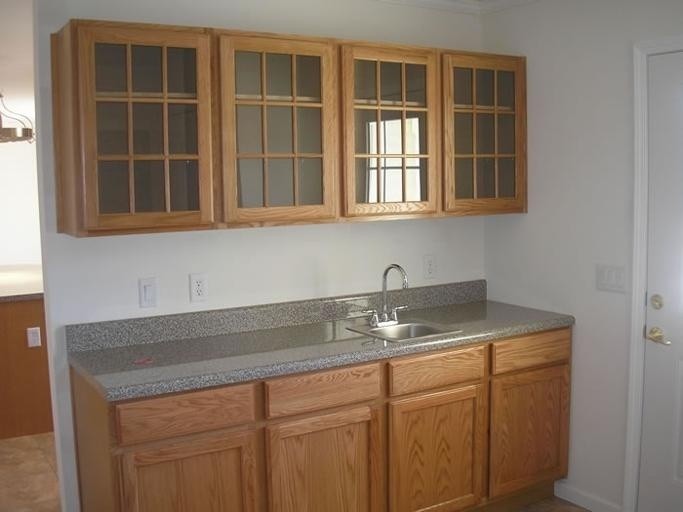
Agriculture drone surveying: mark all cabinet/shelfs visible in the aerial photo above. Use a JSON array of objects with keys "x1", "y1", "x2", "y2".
[
  {"x1": 340, "y1": 38, "x2": 441, "y2": 221},
  {"x1": 442, "y1": 49, "x2": 527, "y2": 217},
  {"x1": 261, "y1": 363, "x2": 383, "y2": 510},
  {"x1": 218, "y1": 27, "x2": 341, "y2": 228},
  {"x1": 50, "y1": 18, "x2": 215, "y2": 238},
  {"x1": 115, "y1": 384, "x2": 260, "y2": 511},
  {"x1": 385, "y1": 341, "x2": 489, "y2": 509},
  {"x1": 488, "y1": 330, "x2": 570, "y2": 500}
]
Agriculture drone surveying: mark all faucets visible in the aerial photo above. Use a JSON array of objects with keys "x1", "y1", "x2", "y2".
[{"x1": 381, "y1": 263, "x2": 409, "y2": 320}]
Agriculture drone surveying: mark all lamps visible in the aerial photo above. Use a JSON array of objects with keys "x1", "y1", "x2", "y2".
[{"x1": 0, "y1": 94, "x2": 35, "y2": 143}]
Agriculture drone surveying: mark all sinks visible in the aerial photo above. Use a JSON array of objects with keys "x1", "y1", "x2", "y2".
[{"x1": 368, "y1": 321, "x2": 463, "y2": 343}]
